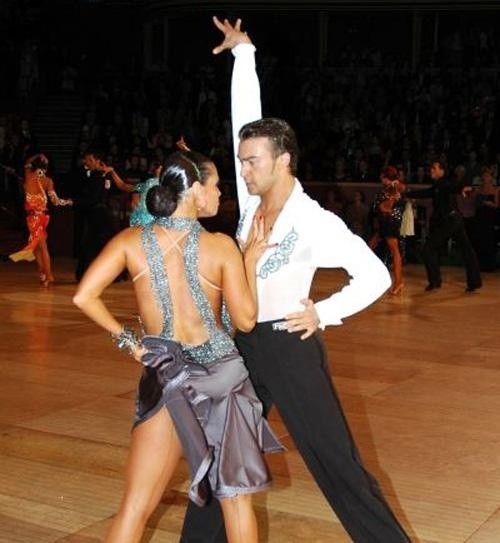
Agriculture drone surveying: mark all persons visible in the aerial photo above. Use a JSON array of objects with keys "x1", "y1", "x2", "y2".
[
  {"x1": 366, "y1": 159, "x2": 500, "y2": 295},
  {"x1": 9, "y1": 153, "x2": 73, "y2": 288},
  {"x1": 73, "y1": 151, "x2": 282, "y2": 543},
  {"x1": 177, "y1": 16, "x2": 413, "y2": 543},
  {"x1": 72, "y1": 136, "x2": 191, "y2": 283},
  {"x1": 1, "y1": 47, "x2": 500, "y2": 273}
]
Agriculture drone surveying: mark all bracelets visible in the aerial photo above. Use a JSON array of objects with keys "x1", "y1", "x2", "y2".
[{"x1": 116, "y1": 325, "x2": 139, "y2": 351}]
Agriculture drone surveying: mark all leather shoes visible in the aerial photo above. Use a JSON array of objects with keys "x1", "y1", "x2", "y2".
[
  {"x1": 465, "y1": 281, "x2": 482, "y2": 292},
  {"x1": 425, "y1": 282, "x2": 442, "y2": 292}
]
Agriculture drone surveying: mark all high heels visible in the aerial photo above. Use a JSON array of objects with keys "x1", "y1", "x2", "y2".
[
  {"x1": 390, "y1": 282, "x2": 404, "y2": 294},
  {"x1": 39, "y1": 273, "x2": 54, "y2": 288}
]
